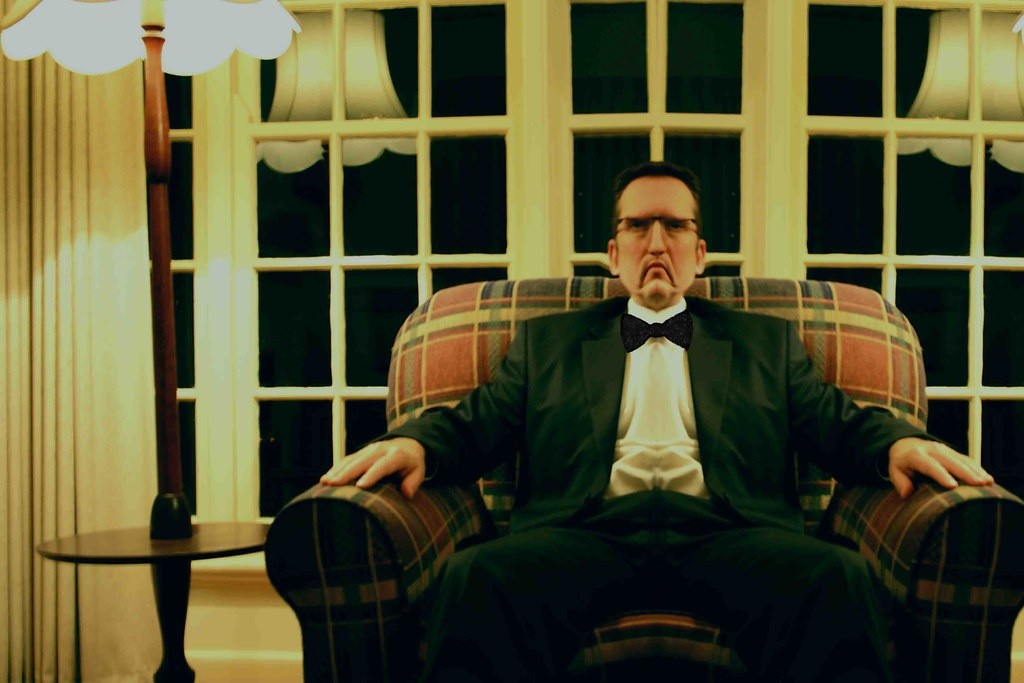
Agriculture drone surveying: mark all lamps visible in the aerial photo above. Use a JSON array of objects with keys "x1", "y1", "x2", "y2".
[
  {"x1": 256, "y1": 10, "x2": 416, "y2": 171},
  {"x1": 0, "y1": 0, "x2": 303, "y2": 683},
  {"x1": 898, "y1": 10, "x2": 1024, "y2": 174}
]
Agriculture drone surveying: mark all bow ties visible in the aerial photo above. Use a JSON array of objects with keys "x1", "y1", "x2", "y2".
[{"x1": 620, "y1": 309, "x2": 694, "y2": 353}]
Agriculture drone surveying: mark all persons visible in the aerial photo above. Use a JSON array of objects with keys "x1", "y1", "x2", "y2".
[{"x1": 321, "y1": 163, "x2": 993, "y2": 683}]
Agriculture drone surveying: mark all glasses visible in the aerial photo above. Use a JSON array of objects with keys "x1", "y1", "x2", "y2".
[{"x1": 613, "y1": 216, "x2": 702, "y2": 237}]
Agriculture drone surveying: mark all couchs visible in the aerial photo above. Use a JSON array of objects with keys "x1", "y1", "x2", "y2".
[{"x1": 265, "y1": 276, "x2": 1024, "y2": 683}]
